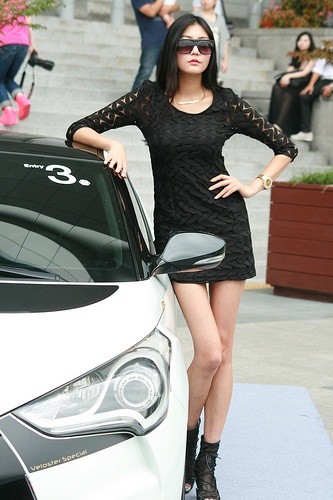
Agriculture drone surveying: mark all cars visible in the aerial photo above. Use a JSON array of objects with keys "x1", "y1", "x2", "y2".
[{"x1": 1, "y1": 128, "x2": 228, "y2": 483}]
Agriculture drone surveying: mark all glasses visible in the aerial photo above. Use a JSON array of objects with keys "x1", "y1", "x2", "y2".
[{"x1": 175, "y1": 39, "x2": 213, "y2": 55}]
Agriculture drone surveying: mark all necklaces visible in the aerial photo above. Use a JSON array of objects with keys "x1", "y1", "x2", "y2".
[{"x1": 178, "y1": 86, "x2": 205, "y2": 105}]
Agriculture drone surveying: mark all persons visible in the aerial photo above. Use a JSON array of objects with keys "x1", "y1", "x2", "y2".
[
  {"x1": 131, "y1": 0, "x2": 180, "y2": 90},
  {"x1": 195, "y1": 0, "x2": 230, "y2": 78},
  {"x1": 66, "y1": 13, "x2": 298, "y2": 500},
  {"x1": 268, "y1": 31, "x2": 333, "y2": 142},
  {"x1": 0, "y1": 0, "x2": 33, "y2": 125}
]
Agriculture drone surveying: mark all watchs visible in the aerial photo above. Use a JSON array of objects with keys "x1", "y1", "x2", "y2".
[{"x1": 257, "y1": 173, "x2": 272, "y2": 190}]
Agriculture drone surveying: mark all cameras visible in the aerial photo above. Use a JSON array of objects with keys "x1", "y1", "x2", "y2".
[{"x1": 28, "y1": 50, "x2": 54, "y2": 71}]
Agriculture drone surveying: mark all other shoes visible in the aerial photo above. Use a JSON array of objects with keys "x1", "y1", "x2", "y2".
[{"x1": 291, "y1": 131, "x2": 313, "y2": 142}]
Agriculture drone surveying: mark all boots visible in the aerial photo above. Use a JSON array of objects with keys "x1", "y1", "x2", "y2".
[
  {"x1": 15, "y1": 94, "x2": 30, "y2": 120},
  {"x1": 1, "y1": 108, "x2": 16, "y2": 126},
  {"x1": 184, "y1": 418, "x2": 201, "y2": 494},
  {"x1": 194, "y1": 435, "x2": 222, "y2": 500}
]
